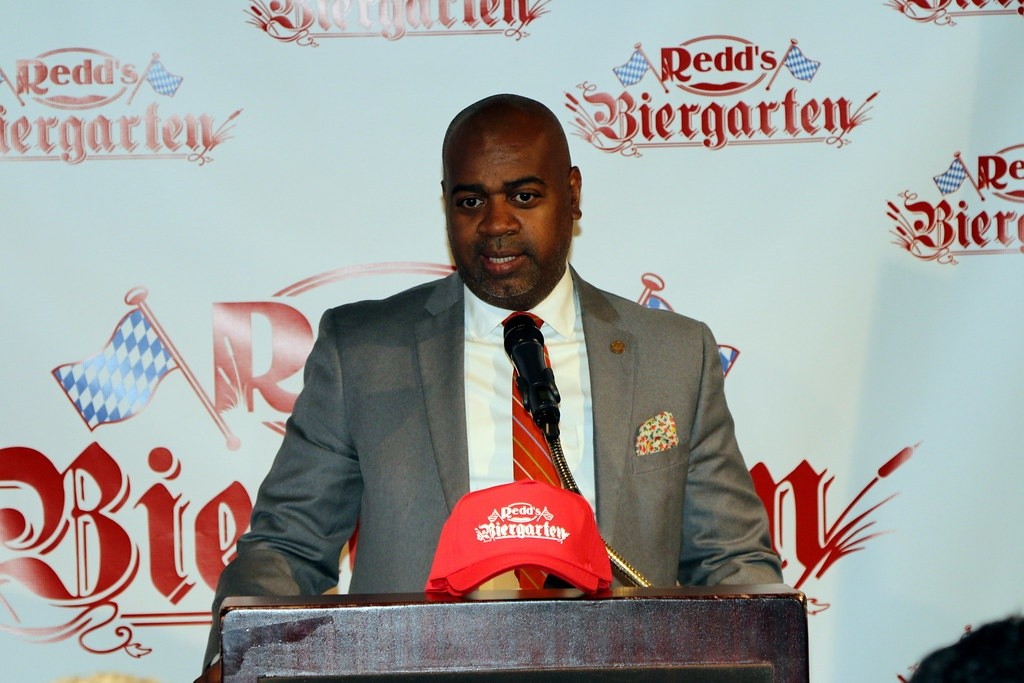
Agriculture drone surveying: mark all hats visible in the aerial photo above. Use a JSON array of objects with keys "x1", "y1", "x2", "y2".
[{"x1": 424, "y1": 479, "x2": 613, "y2": 594}]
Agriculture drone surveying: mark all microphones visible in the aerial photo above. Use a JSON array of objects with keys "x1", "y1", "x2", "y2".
[{"x1": 503, "y1": 315, "x2": 561, "y2": 442}]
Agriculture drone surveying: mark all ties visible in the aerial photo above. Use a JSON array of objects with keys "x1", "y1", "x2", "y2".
[{"x1": 500, "y1": 312, "x2": 562, "y2": 591}]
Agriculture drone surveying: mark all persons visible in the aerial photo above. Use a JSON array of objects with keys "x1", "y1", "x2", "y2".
[
  {"x1": 907, "y1": 616, "x2": 1024, "y2": 683},
  {"x1": 192, "y1": 93, "x2": 786, "y2": 683}
]
